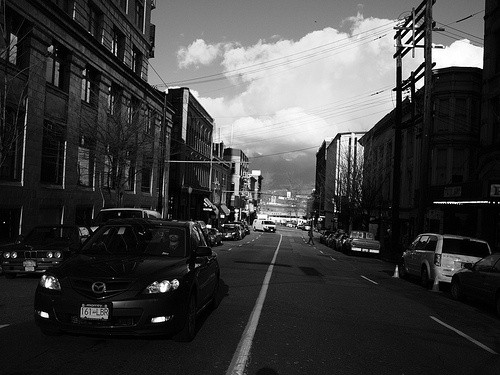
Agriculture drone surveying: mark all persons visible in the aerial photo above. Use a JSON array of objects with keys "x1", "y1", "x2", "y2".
[
  {"x1": 308, "y1": 225, "x2": 315, "y2": 245},
  {"x1": 161, "y1": 229, "x2": 184, "y2": 257}
]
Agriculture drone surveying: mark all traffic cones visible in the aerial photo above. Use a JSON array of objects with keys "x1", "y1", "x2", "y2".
[{"x1": 392, "y1": 264, "x2": 400, "y2": 277}]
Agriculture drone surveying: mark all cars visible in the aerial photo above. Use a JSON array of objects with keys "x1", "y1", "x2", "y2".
[
  {"x1": 204, "y1": 228, "x2": 222, "y2": 246},
  {"x1": 34, "y1": 217, "x2": 219, "y2": 338},
  {"x1": 285, "y1": 222, "x2": 315, "y2": 231},
  {"x1": 0, "y1": 222, "x2": 101, "y2": 276},
  {"x1": 450, "y1": 252, "x2": 500, "y2": 304},
  {"x1": 399, "y1": 232, "x2": 491, "y2": 291},
  {"x1": 221, "y1": 222, "x2": 244, "y2": 240},
  {"x1": 243, "y1": 223, "x2": 250, "y2": 235},
  {"x1": 317, "y1": 228, "x2": 381, "y2": 254}
]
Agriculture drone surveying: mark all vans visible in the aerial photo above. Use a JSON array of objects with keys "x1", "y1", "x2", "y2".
[
  {"x1": 92, "y1": 206, "x2": 162, "y2": 222},
  {"x1": 252, "y1": 219, "x2": 277, "y2": 232}
]
